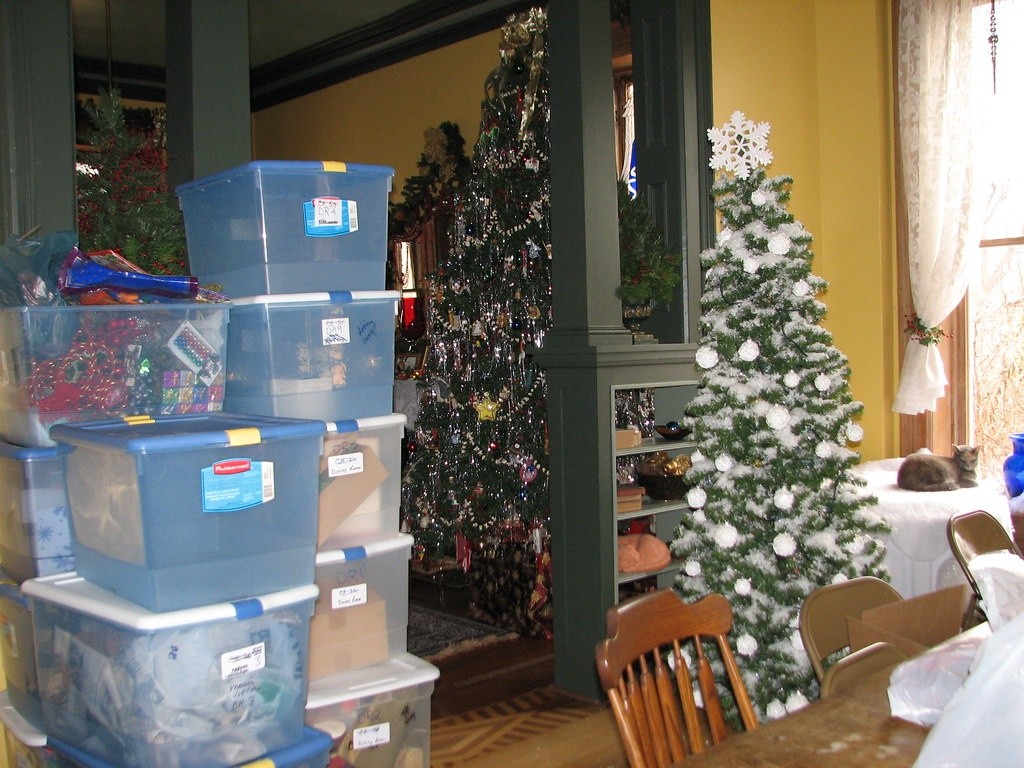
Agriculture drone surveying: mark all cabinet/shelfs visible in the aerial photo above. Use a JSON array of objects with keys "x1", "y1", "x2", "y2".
[{"x1": 608, "y1": 381, "x2": 713, "y2": 608}]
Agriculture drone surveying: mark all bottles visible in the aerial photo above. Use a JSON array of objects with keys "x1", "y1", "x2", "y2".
[{"x1": 1002, "y1": 433, "x2": 1024, "y2": 558}]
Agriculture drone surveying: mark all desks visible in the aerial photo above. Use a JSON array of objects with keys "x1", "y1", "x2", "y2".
[
  {"x1": 670, "y1": 621, "x2": 1002, "y2": 768},
  {"x1": 853, "y1": 458, "x2": 1013, "y2": 601}
]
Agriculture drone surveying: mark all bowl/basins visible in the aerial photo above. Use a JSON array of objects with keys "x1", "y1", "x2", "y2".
[
  {"x1": 638, "y1": 472, "x2": 688, "y2": 500},
  {"x1": 653, "y1": 425, "x2": 692, "y2": 440}
]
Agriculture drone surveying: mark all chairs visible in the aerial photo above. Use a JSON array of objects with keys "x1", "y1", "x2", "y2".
[
  {"x1": 597, "y1": 587, "x2": 757, "y2": 768},
  {"x1": 800, "y1": 575, "x2": 904, "y2": 684},
  {"x1": 946, "y1": 509, "x2": 1024, "y2": 622}
]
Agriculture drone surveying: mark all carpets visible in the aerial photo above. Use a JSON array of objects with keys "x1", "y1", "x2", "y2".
[{"x1": 407, "y1": 595, "x2": 520, "y2": 661}]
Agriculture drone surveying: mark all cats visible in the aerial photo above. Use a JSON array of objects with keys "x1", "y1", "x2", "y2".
[{"x1": 897, "y1": 443, "x2": 980, "y2": 491}]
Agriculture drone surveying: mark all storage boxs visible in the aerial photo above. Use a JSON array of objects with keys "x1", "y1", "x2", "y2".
[
  {"x1": 0, "y1": 160, "x2": 435, "y2": 768},
  {"x1": 846, "y1": 583, "x2": 968, "y2": 657}
]
